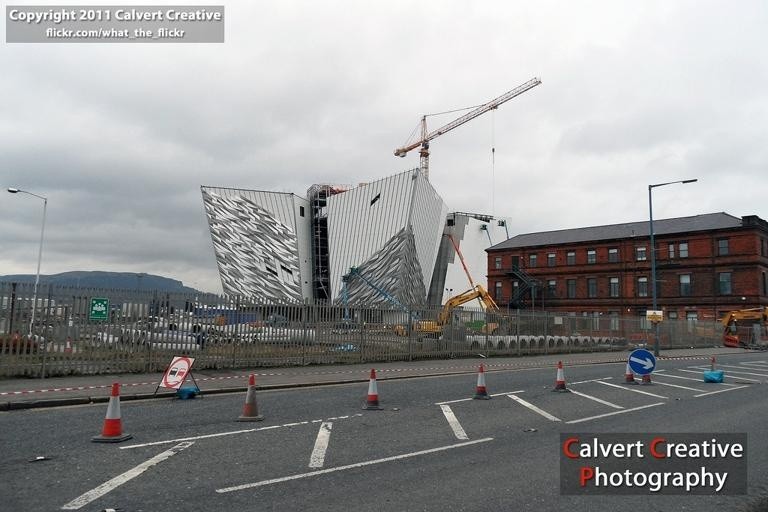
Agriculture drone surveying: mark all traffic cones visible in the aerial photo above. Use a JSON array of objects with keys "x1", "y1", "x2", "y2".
[
  {"x1": 7, "y1": 186, "x2": 48, "y2": 336},
  {"x1": 641, "y1": 373, "x2": 655, "y2": 385},
  {"x1": 471, "y1": 363, "x2": 491, "y2": 401},
  {"x1": 358, "y1": 369, "x2": 381, "y2": 411},
  {"x1": 709, "y1": 357, "x2": 717, "y2": 371},
  {"x1": 549, "y1": 359, "x2": 570, "y2": 394},
  {"x1": 88, "y1": 381, "x2": 132, "y2": 446},
  {"x1": 232, "y1": 372, "x2": 263, "y2": 423},
  {"x1": 621, "y1": 360, "x2": 638, "y2": 386}
]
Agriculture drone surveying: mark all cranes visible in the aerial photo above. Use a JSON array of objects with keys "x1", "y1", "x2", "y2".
[{"x1": 390, "y1": 74, "x2": 543, "y2": 181}]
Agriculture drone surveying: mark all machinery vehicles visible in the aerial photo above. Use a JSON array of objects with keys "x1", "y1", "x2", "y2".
[
  {"x1": 692, "y1": 306, "x2": 767, "y2": 348},
  {"x1": 391, "y1": 283, "x2": 506, "y2": 339}
]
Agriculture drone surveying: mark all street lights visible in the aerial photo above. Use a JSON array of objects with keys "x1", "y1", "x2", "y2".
[
  {"x1": 645, "y1": 177, "x2": 698, "y2": 355},
  {"x1": 448, "y1": 288, "x2": 453, "y2": 299},
  {"x1": 445, "y1": 286, "x2": 449, "y2": 302}
]
